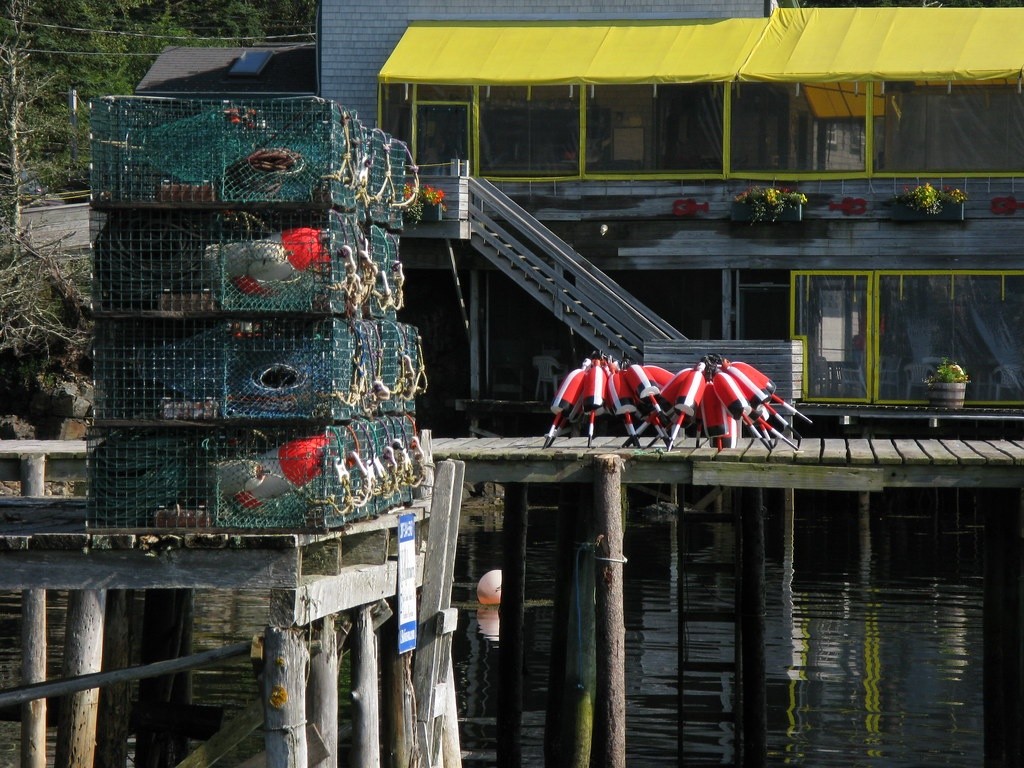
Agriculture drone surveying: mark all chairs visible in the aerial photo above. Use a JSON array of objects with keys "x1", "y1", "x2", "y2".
[
  {"x1": 490, "y1": 338, "x2": 524, "y2": 400},
  {"x1": 812, "y1": 350, "x2": 1024, "y2": 401},
  {"x1": 531, "y1": 355, "x2": 570, "y2": 400}
]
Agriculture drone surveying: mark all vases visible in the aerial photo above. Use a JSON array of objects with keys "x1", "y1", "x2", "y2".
[
  {"x1": 892, "y1": 204, "x2": 965, "y2": 222},
  {"x1": 729, "y1": 204, "x2": 803, "y2": 222},
  {"x1": 928, "y1": 382, "x2": 966, "y2": 408}
]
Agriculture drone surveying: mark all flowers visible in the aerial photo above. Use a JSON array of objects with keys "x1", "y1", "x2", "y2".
[
  {"x1": 733, "y1": 186, "x2": 809, "y2": 223},
  {"x1": 919, "y1": 356, "x2": 973, "y2": 386},
  {"x1": 893, "y1": 182, "x2": 966, "y2": 213}
]
